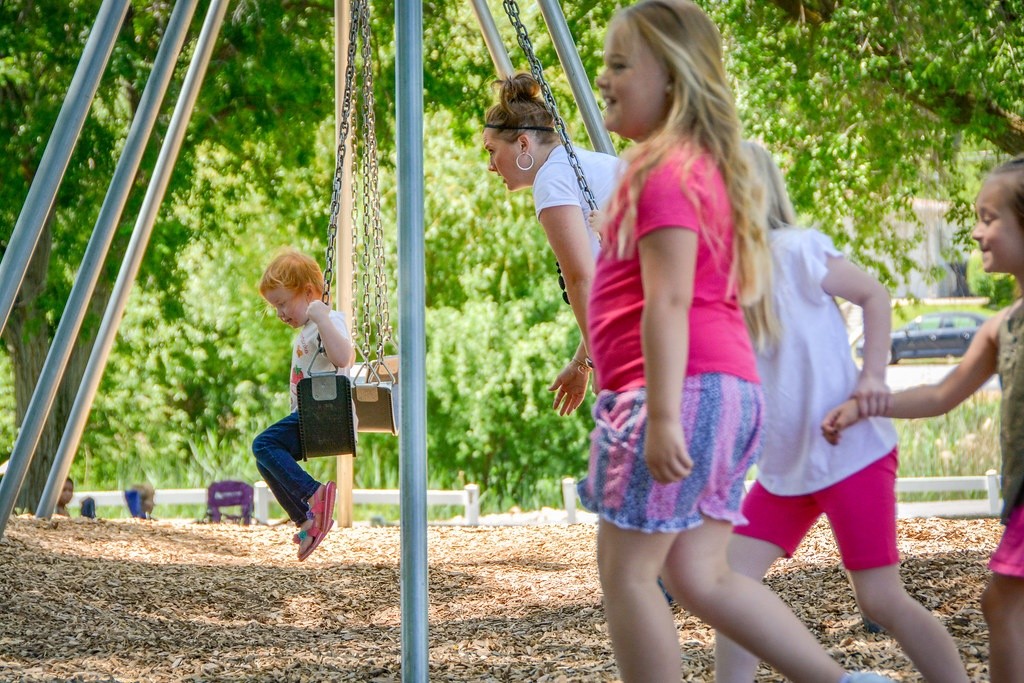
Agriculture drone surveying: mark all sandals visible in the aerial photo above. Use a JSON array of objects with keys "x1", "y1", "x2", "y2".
[
  {"x1": 293, "y1": 518, "x2": 335, "y2": 562},
  {"x1": 311, "y1": 481, "x2": 337, "y2": 534}
]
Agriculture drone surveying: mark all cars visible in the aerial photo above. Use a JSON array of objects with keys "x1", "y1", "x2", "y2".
[{"x1": 853, "y1": 311, "x2": 988, "y2": 363}]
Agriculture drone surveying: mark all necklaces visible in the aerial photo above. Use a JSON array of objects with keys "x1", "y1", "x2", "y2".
[{"x1": 554, "y1": 260, "x2": 569, "y2": 307}]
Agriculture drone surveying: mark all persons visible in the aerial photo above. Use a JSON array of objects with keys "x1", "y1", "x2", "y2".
[
  {"x1": 576, "y1": 1, "x2": 890, "y2": 683},
  {"x1": 819, "y1": 154, "x2": 1024, "y2": 683},
  {"x1": 252, "y1": 250, "x2": 357, "y2": 562},
  {"x1": 53, "y1": 476, "x2": 79, "y2": 518},
  {"x1": 716, "y1": 139, "x2": 970, "y2": 682},
  {"x1": 482, "y1": 73, "x2": 627, "y2": 416}
]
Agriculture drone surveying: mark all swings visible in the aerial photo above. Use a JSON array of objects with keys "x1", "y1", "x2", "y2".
[
  {"x1": 503, "y1": 0, "x2": 602, "y2": 242},
  {"x1": 295, "y1": 0, "x2": 401, "y2": 462}
]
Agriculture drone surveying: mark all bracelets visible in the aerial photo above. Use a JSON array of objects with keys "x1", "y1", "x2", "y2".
[{"x1": 571, "y1": 356, "x2": 592, "y2": 374}]
